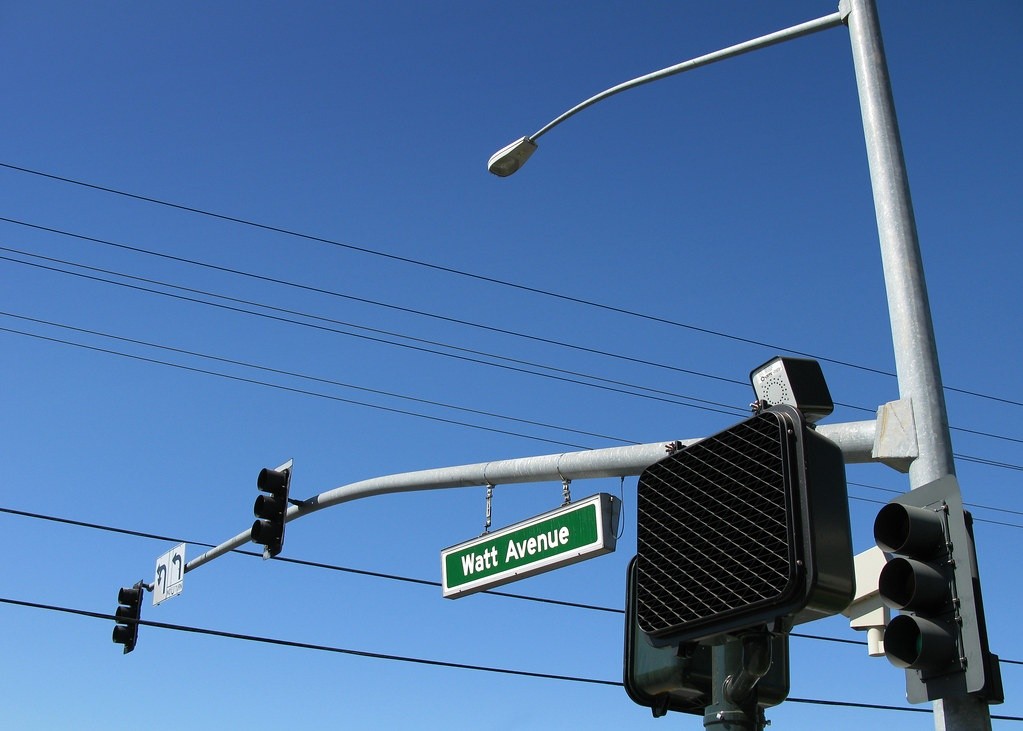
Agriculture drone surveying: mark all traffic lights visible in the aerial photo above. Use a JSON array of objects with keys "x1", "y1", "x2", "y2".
[
  {"x1": 872, "y1": 473, "x2": 986, "y2": 703},
  {"x1": 250, "y1": 458, "x2": 295, "y2": 560},
  {"x1": 112, "y1": 579, "x2": 143, "y2": 654},
  {"x1": 630, "y1": 408, "x2": 862, "y2": 652}
]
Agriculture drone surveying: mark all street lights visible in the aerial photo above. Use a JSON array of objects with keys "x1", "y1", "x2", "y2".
[{"x1": 486, "y1": 1, "x2": 1008, "y2": 731}]
